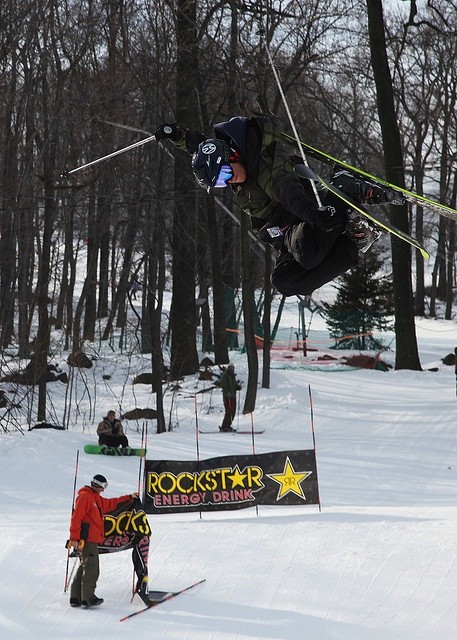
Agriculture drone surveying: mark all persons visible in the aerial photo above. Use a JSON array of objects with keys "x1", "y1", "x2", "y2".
[
  {"x1": 68, "y1": 474, "x2": 139, "y2": 610},
  {"x1": 156, "y1": 116, "x2": 406, "y2": 296},
  {"x1": 97, "y1": 411, "x2": 127, "y2": 448},
  {"x1": 220, "y1": 363, "x2": 241, "y2": 432}
]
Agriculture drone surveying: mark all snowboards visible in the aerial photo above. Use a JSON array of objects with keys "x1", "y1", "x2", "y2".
[{"x1": 85, "y1": 445, "x2": 147, "y2": 456}]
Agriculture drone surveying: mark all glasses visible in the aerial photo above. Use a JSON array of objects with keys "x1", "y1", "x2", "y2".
[
  {"x1": 214, "y1": 162, "x2": 234, "y2": 188},
  {"x1": 93, "y1": 478, "x2": 108, "y2": 489}
]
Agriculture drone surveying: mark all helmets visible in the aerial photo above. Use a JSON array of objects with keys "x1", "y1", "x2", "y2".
[
  {"x1": 191, "y1": 139, "x2": 240, "y2": 194},
  {"x1": 228, "y1": 363, "x2": 234, "y2": 368}
]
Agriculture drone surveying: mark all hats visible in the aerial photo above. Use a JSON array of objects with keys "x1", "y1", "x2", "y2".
[{"x1": 108, "y1": 411, "x2": 115, "y2": 417}]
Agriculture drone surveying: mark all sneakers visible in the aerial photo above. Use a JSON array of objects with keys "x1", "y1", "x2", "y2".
[
  {"x1": 126, "y1": 446, "x2": 131, "y2": 449},
  {"x1": 82, "y1": 599, "x2": 103, "y2": 609},
  {"x1": 222, "y1": 426, "x2": 237, "y2": 432},
  {"x1": 101, "y1": 444, "x2": 107, "y2": 447},
  {"x1": 349, "y1": 216, "x2": 375, "y2": 248},
  {"x1": 70, "y1": 598, "x2": 82, "y2": 607},
  {"x1": 330, "y1": 171, "x2": 388, "y2": 205}
]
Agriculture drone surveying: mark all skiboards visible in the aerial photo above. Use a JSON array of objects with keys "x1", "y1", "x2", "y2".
[
  {"x1": 199, "y1": 429, "x2": 265, "y2": 435},
  {"x1": 282, "y1": 131, "x2": 456, "y2": 258}
]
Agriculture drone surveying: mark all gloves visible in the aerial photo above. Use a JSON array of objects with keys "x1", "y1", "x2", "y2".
[
  {"x1": 112, "y1": 428, "x2": 119, "y2": 434},
  {"x1": 309, "y1": 208, "x2": 342, "y2": 232},
  {"x1": 237, "y1": 385, "x2": 242, "y2": 391},
  {"x1": 153, "y1": 121, "x2": 189, "y2": 141},
  {"x1": 226, "y1": 392, "x2": 231, "y2": 399}
]
